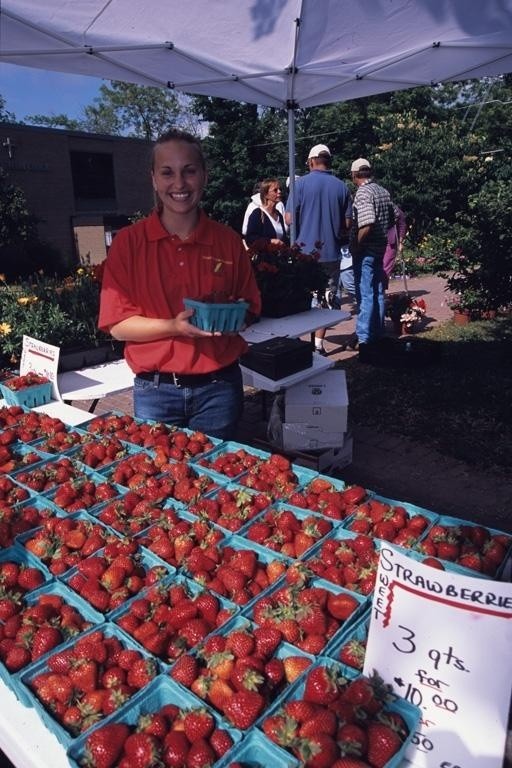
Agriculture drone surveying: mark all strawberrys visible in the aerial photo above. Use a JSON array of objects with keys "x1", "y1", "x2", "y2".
[
  {"x1": 0, "y1": 370, "x2": 512, "y2": 768},
  {"x1": 195, "y1": 287, "x2": 238, "y2": 303}
]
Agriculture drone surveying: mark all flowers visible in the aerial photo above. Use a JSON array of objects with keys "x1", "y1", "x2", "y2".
[
  {"x1": 0, "y1": 268, "x2": 112, "y2": 363},
  {"x1": 244, "y1": 236, "x2": 323, "y2": 300},
  {"x1": 384, "y1": 293, "x2": 426, "y2": 328}
]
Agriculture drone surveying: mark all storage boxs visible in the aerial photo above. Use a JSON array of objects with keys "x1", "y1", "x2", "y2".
[{"x1": 254, "y1": 372, "x2": 356, "y2": 476}]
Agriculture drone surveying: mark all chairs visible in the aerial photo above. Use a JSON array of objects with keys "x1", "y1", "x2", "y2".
[{"x1": 16, "y1": 333, "x2": 105, "y2": 411}]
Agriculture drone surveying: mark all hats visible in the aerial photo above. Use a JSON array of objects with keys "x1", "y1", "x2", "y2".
[
  {"x1": 351, "y1": 158, "x2": 371, "y2": 172},
  {"x1": 305, "y1": 144, "x2": 331, "y2": 166},
  {"x1": 286, "y1": 175, "x2": 302, "y2": 188}
]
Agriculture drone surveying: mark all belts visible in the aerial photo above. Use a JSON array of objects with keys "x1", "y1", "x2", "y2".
[{"x1": 135, "y1": 359, "x2": 242, "y2": 389}]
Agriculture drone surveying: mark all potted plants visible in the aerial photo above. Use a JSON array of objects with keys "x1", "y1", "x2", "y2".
[{"x1": 442, "y1": 181, "x2": 509, "y2": 327}]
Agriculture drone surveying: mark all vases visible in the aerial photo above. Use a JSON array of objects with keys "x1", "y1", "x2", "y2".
[
  {"x1": 54, "y1": 343, "x2": 119, "y2": 375},
  {"x1": 393, "y1": 320, "x2": 414, "y2": 335},
  {"x1": 256, "y1": 290, "x2": 312, "y2": 318}
]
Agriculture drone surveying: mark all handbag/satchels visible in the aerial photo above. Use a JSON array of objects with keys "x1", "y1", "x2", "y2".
[
  {"x1": 240, "y1": 336, "x2": 312, "y2": 381},
  {"x1": 359, "y1": 336, "x2": 429, "y2": 369}
]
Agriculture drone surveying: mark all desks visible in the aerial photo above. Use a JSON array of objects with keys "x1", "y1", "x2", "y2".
[
  {"x1": 0, "y1": 392, "x2": 512, "y2": 768},
  {"x1": 239, "y1": 307, "x2": 352, "y2": 423}
]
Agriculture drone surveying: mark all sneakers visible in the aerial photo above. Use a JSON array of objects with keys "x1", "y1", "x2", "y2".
[{"x1": 315, "y1": 347, "x2": 329, "y2": 357}]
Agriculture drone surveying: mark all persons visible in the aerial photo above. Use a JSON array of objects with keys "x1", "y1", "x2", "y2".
[
  {"x1": 241, "y1": 144, "x2": 408, "y2": 357},
  {"x1": 98, "y1": 128, "x2": 262, "y2": 441}
]
